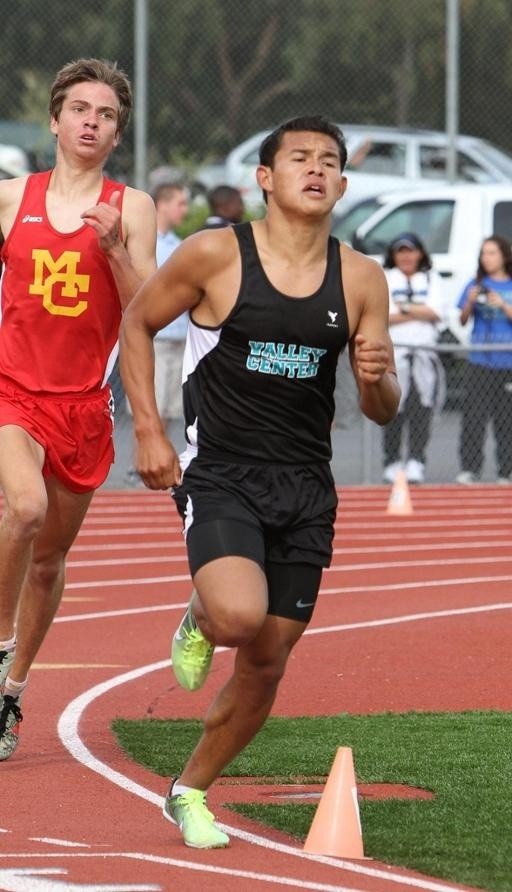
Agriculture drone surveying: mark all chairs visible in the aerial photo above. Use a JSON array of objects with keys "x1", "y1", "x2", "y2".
[
  {"x1": 1, "y1": 686, "x2": 25, "y2": 760},
  {"x1": 171, "y1": 589, "x2": 216, "y2": 692},
  {"x1": 162, "y1": 778, "x2": 231, "y2": 849},
  {"x1": 381, "y1": 457, "x2": 425, "y2": 483},
  {"x1": 493, "y1": 473, "x2": 512, "y2": 487},
  {"x1": 453, "y1": 468, "x2": 483, "y2": 486},
  {"x1": 0, "y1": 643, "x2": 17, "y2": 687}
]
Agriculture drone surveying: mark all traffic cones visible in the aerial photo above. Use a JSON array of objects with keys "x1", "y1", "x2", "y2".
[{"x1": 299, "y1": 745, "x2": 374, "y2": 862}]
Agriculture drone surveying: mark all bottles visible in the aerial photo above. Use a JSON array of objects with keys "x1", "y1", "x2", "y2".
[
  {"x1": 0, "y1": 144, "x2": 33, "y2": 179},
  {"x1": 389, "y1": 235, "x2": 419, "y2": 252}
]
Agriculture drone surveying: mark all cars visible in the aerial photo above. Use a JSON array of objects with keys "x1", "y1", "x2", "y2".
[
  {"x1": 204, "y1": 123, "x2": 512, "y2": 250},
  {"x1": 323, "y1": 180, "x2": 512, "y2": 367}
]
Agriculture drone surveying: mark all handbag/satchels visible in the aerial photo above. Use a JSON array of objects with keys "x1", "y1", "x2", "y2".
[{"x1": 399, "y1": 301, "x2": 412, "y2": 317}]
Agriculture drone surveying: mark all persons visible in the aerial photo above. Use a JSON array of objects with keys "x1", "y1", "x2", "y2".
[
  {"x1": 113, "y1": 114, "x2": 402, "y2": 854},
  {"x1": 0, "y1": 58, "x2": 164, "y2": 762},
  {"x1": 1, "y1": 141, "x2": 32, "y2": 180},
  {"x1": 195, "y1": 184, "x2": 245, "y2": 229},
  {"x1": 380, "y1": 230, "x2": 452, "y2": 486},
  {"x1": 452, "y1": 235, "x2": 511, "y2": 486},
  {"x1": 123, "y1": 183, "x2": 195, "y2": 488}
]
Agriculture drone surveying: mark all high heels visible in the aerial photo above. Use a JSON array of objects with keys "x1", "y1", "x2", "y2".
[{"x1": 299, "y1": 745, "x2": 374, "y2": 862}]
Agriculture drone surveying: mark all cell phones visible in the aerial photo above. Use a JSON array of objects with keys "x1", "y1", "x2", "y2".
[{"x1": 477, "y1": 285, "x2": 490, "y2": 295}]
